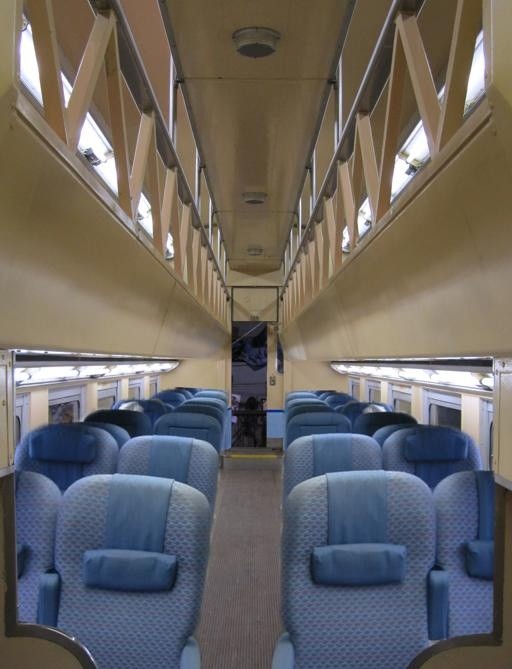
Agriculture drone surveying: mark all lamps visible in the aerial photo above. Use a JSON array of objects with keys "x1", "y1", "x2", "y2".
[
  {"x1": 247, "y1": 248, "x2": 263, "y2": 255},
  {"x1": 242, "y1": 192, "x2": 268, "y2": 204},
  {"x1": 231, "y1": 25, "x2": 283, "y2": 58}
]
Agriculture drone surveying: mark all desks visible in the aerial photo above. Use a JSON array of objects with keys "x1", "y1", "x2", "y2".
[{"x1": 232, "y1": 410, "x2": 266, "y2": 447}]
